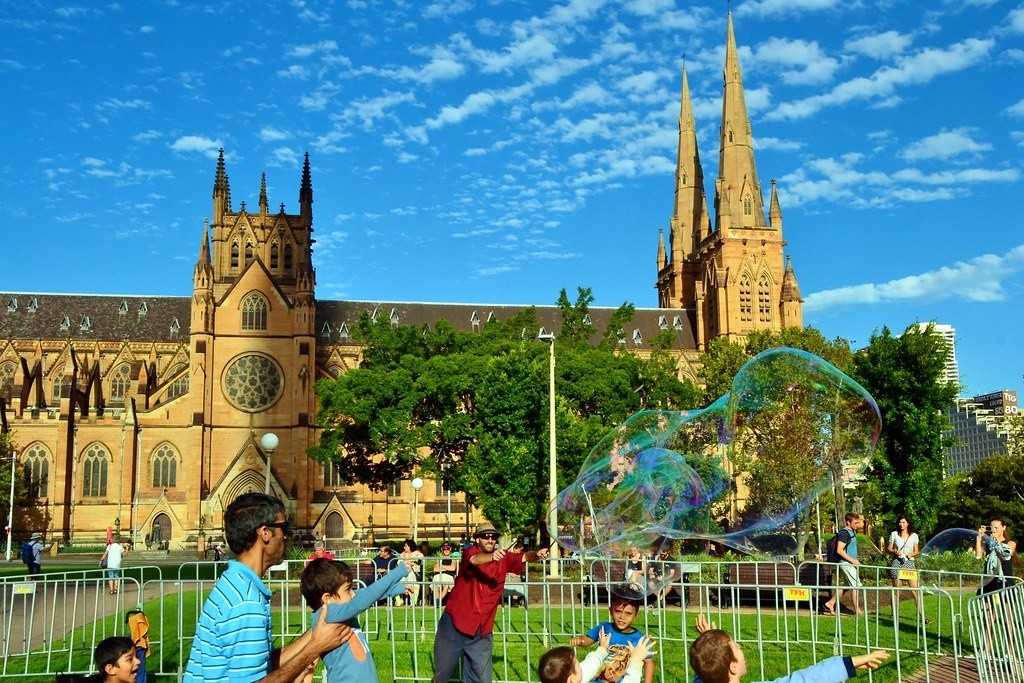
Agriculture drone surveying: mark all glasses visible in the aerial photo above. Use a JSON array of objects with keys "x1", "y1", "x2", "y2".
[
  {"x1": 254, "y1": 520, "x2": 290, "y2": 536},
  {"x1": 476, "y1": 533, "x2": 499, "y2": 540},
  {"x1": 443, "y1": 547, "x2": 450, "y2": 551},
  {"x1": 660, "y1": 552, "x2": 667, "y2": 558}
]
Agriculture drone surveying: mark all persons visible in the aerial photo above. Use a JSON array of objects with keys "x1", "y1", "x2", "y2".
[
  {"x1": 687, "y1": 613, "x2": 892, "y2": 683},
  {"x1": 182, "y1": 492, "x2": 355, "y2": 683},
  {"x1": 294, "y1": 657, "x2": 320, "y2": 683},
  {"x1": 975, "y1": 519, "x2": 1018, "y2": 659},
  {"x1": 824, "y1": 513, "x2": 865, "y2": 617},
  {"x1": 99, "y1": 534, "x2": 130, "y2": 595},
  {"x1": 27, "y1": 532, "x2": 54, "y2": 593},
  {"x1": 300, "y1": 557, "x2": 414, "y2": 683},
  {"x1": 94, "y1": 636, "x2": 141, "y2": 682},
  {"x1": 214, "y1": 545, "x2": 226, "y2": 561},
  {"x1": 430, "y1": 524, "x2": 549, "y2": 683},
  {"x1": 568, "y1": 588, "x2": 654, "y2": 683},
  {"x1": 538, "y1": 625, "x2": 658, "y2": 683},
  {"x1": 887, "y1": 515, "x2": 933, "y2": 624},
  {"x1": 624, "y1": 544, "x2": 676, "y2": 608},
  {"x1": 299, "y1": 530, "x2": 524, "y2": 608}
]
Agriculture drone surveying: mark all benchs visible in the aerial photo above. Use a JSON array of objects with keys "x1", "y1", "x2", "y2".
[
  {"x1": 585, "y1": 562, "x2": 691, "y2": 607},
  {"x1": 723, "y1": 564, "x2": 832, "y2": 610},
  {"x1": 427, "y1": 572, "x2": 527, "y2": 607},
  {"x1": 345, "y1": 563, "x2": 374, "y2": 589}
]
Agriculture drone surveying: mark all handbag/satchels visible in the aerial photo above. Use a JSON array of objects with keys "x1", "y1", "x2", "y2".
[
  {"x1": 885, "y1": 563, "x2": 893, "y2": 579},
  {"x1": 101, "y1": 558, "x2": 107, "y2": 568}
]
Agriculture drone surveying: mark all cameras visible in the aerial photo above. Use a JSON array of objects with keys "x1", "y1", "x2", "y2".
[{"x1": 985, "y1": 526, "x2": 996, "y2": 532}]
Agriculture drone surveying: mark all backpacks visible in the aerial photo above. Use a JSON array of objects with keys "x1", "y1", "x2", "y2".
[
  {"x1": 22, "y1": 541, "x2": 40, "y2": 564},
  {"x1": 826, "y1": 529, "x2": 851, "y2": 563}
]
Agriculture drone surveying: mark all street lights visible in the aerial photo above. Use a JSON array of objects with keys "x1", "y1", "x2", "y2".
[
  {"x1": 1, "y1": 456, "x2": 16, "y2": 561},
  {"x1": 539, "y1": 334, "x2": 559, "y2": 578},
  {"x1": 413, "y1": 478, "x2": 423, "y2": 541},
  {"x1": 260, "y1": 432, "x2": 280, "y2": 496}
]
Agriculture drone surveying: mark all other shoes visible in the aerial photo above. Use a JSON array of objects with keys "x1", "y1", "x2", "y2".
[
  {"x1": 999, "y1": 654, "x2": 1014, "y2": 662},
  {"x1": 920, "y1": 619, "x2": 932, "y2": 625},
  {"x1": 889, "y1": 614, "x2": 894, "y2": 619},
  {"x1": 970, "y1": 650, "x2": 991, "y2": 659},
  {"x1": 114, "y1": 588, "x2": 118, "y2": 594},
  {"x1": 410, "y1": 604, "x2": 417, "y2": 608},
  {"x1": 395, "y1": 599, "x2": 404, "y2": 607},
  {"x1": 109, "y1": 590, "x2": 114, "y2": 594},
  {"x1": 660, "y1": 599, "x2": 666, "y2": 607},
  {"x1": 651, "y1": 600, "x2": 657, "y2": 607}
]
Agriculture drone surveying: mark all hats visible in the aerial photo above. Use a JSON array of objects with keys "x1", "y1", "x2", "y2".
[
  {"x1": 440, "y1": 542, "x2": 452, "y2": 552},
  {"x1": 473, "y1": 521, "x2": 501, "y2": 541},
  {"x1": 30, "y1": 533, "x2": 41, "y2": 539}
]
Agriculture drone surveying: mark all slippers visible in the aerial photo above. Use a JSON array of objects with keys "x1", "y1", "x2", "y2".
[
  {"x1": 823, "y1": 603, "x2": 835, "y2": 616},
  {"x1": 852, "y1": 609, "x2": 865, "y2": 616}
]
